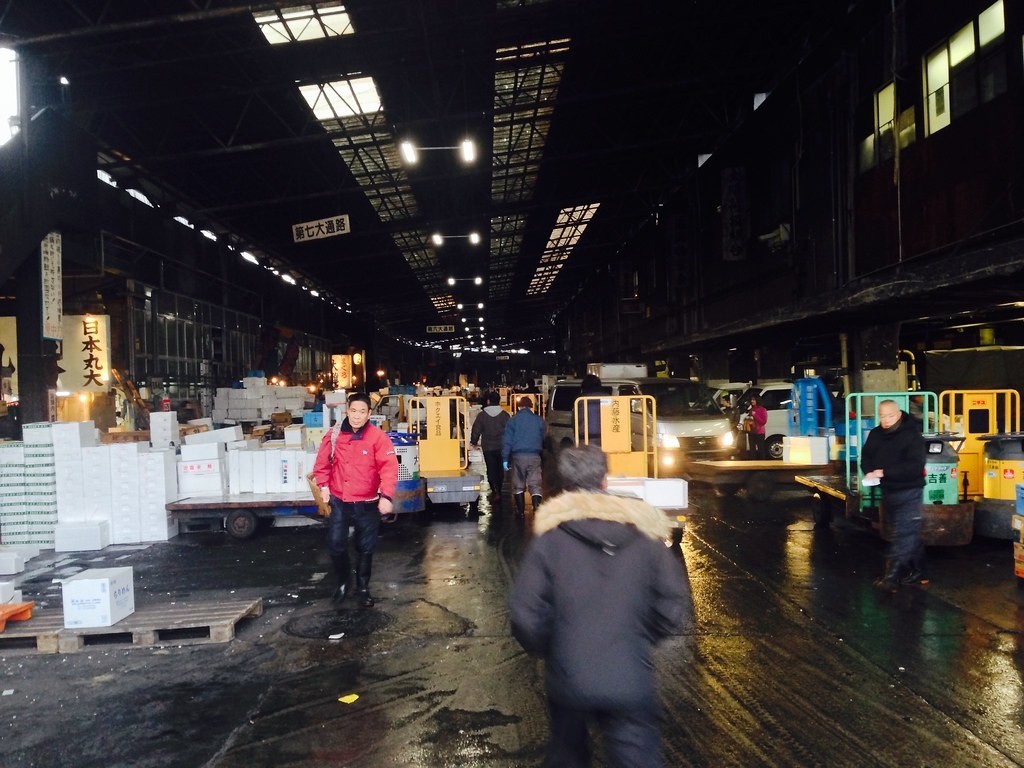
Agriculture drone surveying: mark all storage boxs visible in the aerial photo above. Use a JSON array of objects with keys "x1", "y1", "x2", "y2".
[
  {"x1": 607, "y1": 475, "x2": 688, "y2": 509},
  {"x1": 782, "y1": 437, "x2": 828, "y2": 464},
  {"x1": 1011, "y1": 483, "x2": 1024, "y2": 579},
  {"x1": 0, "y1": 582, "x2": 23, "y2": 604},
  {"x1": 466, "y1": 405, "x2": 483, "y2": 462},
  {"x1": 0, "y1": 375, "x2": 426, "y2": 575},
  {"x1": 62, "y1": 566, "x2": 134, "y2": 628}
]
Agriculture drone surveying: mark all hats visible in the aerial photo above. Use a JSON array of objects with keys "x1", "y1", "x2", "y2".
[
  {"x1": 486, "y1": 392, "x2": 500, "y2": 402},
  {"x1": 518, "y1": 397, "x2": 533, "y2": 408}
]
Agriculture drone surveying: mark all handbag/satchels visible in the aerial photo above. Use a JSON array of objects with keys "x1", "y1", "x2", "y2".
[
  {"x1": 743, "y1": 411, "x2": 756, "y2": 433},
  {"x1": 306, "y1": 473, "x2": 332, "y2": 516}
]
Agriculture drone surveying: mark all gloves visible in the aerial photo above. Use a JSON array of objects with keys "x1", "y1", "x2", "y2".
[{"x1": 502, "y1": 462, "x2": 509, "y2": 471}]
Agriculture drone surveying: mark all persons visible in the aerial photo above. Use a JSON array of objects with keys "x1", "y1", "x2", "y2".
[
  {"x1": 722, "y1": 394, "x2": 740, "y2": 435},
  {"x1": 508, "y1": 443, "x2": 695, "y2": 768},
  {"x1": 578, "y1": 374, "x2": 610, "y2": 446},
  {"x1": 470, "y1": 392, "x2": 510, "y2": 501},
  {"x1": 313, "y1": 393, "x2": 398, "y2": 607},
  {"x1": 861, "y1": 399, "x2": 926, "y2": 590},
  {"x1": 176, "y1": 400, "x2": 198, "y2": 424},
  {"x1": 908, "y1": 389, "x2": 923, "y2": 419},
  {"x1": 502, "y1": 397, "x2": 547, "y2": 519},
  {"x1": 744, "y1": 393, "x2": 767, "y2": 459}
]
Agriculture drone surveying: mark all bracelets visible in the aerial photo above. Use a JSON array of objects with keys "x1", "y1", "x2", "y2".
[{"x1": 382, "y1": 495, "x2": 391, "y2": 502}]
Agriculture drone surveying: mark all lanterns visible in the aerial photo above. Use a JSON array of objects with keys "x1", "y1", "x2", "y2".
[{"x1": 353, "y1": 353, "x2": 361, "y2": 365}]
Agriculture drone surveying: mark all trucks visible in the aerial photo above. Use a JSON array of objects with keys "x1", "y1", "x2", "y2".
[{"x1": 688, "y1": 381, "x2": 795, "y2": 460}]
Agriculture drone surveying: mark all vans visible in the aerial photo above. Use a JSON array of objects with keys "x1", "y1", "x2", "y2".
[{"x1": 545, "y1": 374, "x2": 740, "y2": 475}]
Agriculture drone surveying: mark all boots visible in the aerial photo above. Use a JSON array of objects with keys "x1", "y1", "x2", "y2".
[
  {"x1": 356, "y1": 552, "x2": 374, "y2": 606},
  {"x1": 488, "y1": 480, "x2": 503, "y2": 504},
  {"x1": 877, "y1": 559, "x2": 902, "y2": 593},
  {"x1": 902, "y1": 552, "x2": 930, "y2": 586},
  {"x1": 331, "y1": 549, "x2": 352, "y2": 603},
  {"x1": 514, "y1": 493, "x2": 525, "y2": 518},
  {"x1": 532, "y1": 495, "x2": 542, "y2": 518}
]
